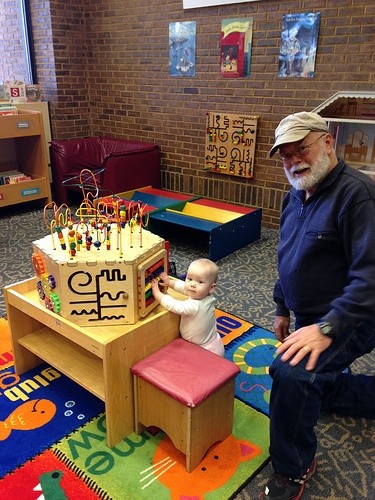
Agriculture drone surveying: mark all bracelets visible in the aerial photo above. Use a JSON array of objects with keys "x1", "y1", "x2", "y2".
[{"x1": 319, "y1": 321, "x2": 333, "y2": 338}]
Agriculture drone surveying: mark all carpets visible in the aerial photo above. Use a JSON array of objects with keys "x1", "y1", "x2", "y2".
[{"x1": 0, "y1": 307, "x2": 350, "y2": 500}]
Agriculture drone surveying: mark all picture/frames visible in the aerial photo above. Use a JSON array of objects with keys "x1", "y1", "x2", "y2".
[
  {"x1": 5, "y1": 81, "x2": 26, "y2": 102},
  {"x1": 26, "y1": 85, "x2": 42, "y2": 102},
  {"x1": 0, "y1": 85, "x2": 10, "y2": 101}
]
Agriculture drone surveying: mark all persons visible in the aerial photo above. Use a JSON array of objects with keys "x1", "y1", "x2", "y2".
[
  {"x1": 258, "y1": 111, "x2": 375, "y2": 500},
  {"x1": 151, "y1": 258, "x2": 224, "y2": 357}
]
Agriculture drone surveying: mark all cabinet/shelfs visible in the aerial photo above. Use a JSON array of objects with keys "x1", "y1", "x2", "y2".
[
  {"x1": 0, "y1": 102, "x2": 53, "y2": 209},
  {"x1": 5, "y1": 272, "x2": 186, "y2": 449}
]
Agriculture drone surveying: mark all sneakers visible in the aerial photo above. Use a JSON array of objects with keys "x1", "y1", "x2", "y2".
[{"x1": 258, "y1": 456, "x2": 317, "y2": 500}]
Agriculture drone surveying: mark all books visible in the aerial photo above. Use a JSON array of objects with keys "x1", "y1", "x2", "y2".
[{"x1": 0, "y1": 170, "x2": 31, "y2": 185}]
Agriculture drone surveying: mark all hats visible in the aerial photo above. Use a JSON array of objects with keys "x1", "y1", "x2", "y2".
[{"x1": 269, "y1": 111, "x2": 330, "y2": 158}]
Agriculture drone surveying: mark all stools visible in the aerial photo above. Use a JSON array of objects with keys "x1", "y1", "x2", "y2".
[{"x1": 131, "y1": 337, "x2": 241, "y2": 473}]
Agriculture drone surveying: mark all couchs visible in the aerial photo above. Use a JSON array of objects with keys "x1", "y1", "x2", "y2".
[{"x1": 48, "y1": 136, "x2": 160, "y2": 208}]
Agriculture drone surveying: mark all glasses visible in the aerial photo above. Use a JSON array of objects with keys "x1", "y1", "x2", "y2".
[{"x1": 277, "y1": 134, "x2": 326, "y2": 163}]
